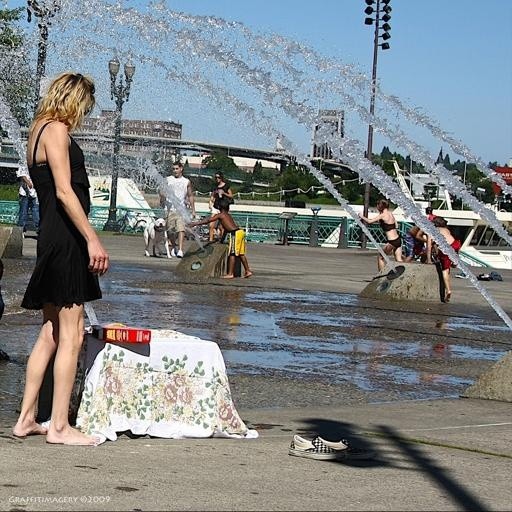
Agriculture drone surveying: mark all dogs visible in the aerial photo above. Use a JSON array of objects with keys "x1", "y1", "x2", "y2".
[{"x1": 143, "y1": 216, "x2": 173, "y2": 259}]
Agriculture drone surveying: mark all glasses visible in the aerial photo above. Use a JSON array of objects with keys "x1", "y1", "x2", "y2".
[{"x1": 215, "y1": 176, "x2": 221, "y2": 179}]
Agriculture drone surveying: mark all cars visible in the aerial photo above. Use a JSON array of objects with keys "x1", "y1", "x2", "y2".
[{"x1": 85, "y1": 166, "x2": 110, "y2": 192}]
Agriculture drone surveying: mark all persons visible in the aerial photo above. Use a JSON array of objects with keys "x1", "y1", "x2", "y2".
[
  {"x1": 15, "y1": 162, "x2": 41, "y2": 234},
  {"x1": 358, "y1": 199, "x2": 461, "y2": 305},
  {"x1": 186, "y1": 196, "x2": 253, "y2": 279},
  {"x1": 10, "y1": 71, "x2": 112, "y2": 447},
  {"x1": 156, "y1": 161, "x2": 197, "y2": 259},
  {"x1": 208, "y1": 171, "x2": 233, "y2": 242}
]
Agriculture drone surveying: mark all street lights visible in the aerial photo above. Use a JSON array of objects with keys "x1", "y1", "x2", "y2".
[
  {"x1": 360, "y1": 0, "x2": 393, "y2": 248},
  {"x1": 190, "y1": 144, "x2": 202, "y2": 174},
  {"x1": 101, "y1": 54, "x2": 135, "y2": 231}
]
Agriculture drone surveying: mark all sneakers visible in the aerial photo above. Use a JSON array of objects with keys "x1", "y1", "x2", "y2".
[
  {"x1": 289, "y1": 435, "x2": 338, "y2": 460},
  {"x1": 318, "y1": 436, "x2": 376, "y2": 458},
  {"x1": 171, "y1": 248, "x2": 176, "y2": 256},
  {"x1": 177, "y1": 249, "x2": 183, "y2": 257}
]
